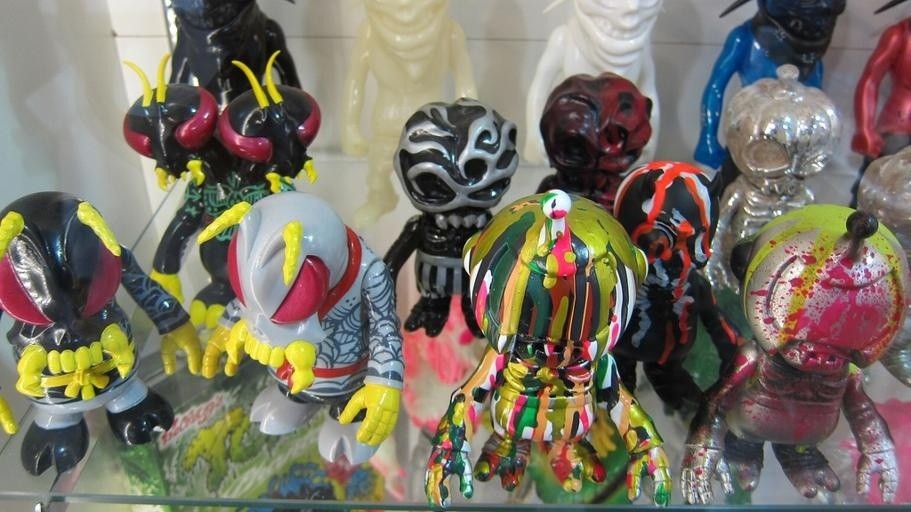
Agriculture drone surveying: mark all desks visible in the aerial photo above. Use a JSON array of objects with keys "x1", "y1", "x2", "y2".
[{"x1": 0, "y1": 160, "x2": 911, "y2": 512}]
